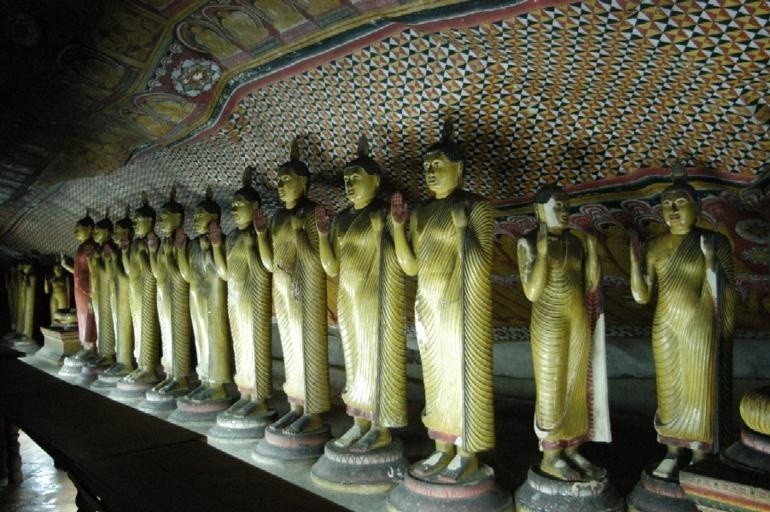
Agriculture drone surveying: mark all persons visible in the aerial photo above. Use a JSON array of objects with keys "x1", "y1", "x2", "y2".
[
  {"x1": 60, "y1": 189, "x2": 234, "y2": 403},
  {"x1": 629, "y1": 182, "x2": 738, "y2": 479},
  {"x1": 209, "y1": 117, "x2": 496, "y2": 483},
  {"x1": 6, "y1": 256, "x2": 70, "y2": 342},
  {"x1": 515, "y1": 188, "x2": 612, "y2": 484}
]
